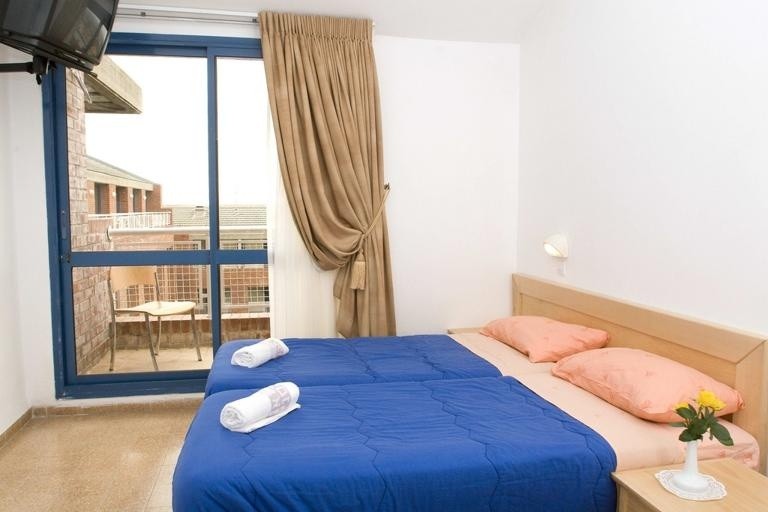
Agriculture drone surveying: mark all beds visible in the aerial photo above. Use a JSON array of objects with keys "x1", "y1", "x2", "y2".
[
  {"x1": 204, "y1": 334, "x2": 558, "y2": 397},
  {"x1": 173, "y1": 371, "x2": 762, "y2": 512}
]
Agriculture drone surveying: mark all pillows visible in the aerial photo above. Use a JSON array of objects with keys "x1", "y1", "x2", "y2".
[
  {"x1": 481, "y1": 314, "x2": 608, "y2": 364},
  {"x1": 552, "y1": 348, "x2": 745, "y2": 424}
]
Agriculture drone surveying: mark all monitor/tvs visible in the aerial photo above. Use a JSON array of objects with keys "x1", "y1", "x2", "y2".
[{"x1": 0, "y1": 0, "x2": 119, "y2": 78}]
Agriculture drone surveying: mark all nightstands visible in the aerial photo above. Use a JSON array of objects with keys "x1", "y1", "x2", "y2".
[
  {"x1": 609, "y1": 458, "x2": 767, "y2": 511},
  {"x1": 448, "y1": 327, "x2": 483, "y2": 334}
]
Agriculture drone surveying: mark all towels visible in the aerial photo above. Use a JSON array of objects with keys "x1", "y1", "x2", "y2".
[
  {"x1": 219, "y1": 382, "x2": 302, "y2": 433},
  {"x1": 232, "y1": 338, "x2": 290, "y2": 369}
]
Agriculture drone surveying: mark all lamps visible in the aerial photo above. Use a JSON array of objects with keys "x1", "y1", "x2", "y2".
[{"x1": 542, "y1": 237, "x2": 568, "y2": 275}]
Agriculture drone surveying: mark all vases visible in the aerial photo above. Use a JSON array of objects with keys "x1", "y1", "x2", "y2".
[{"x1": 674, "y1": 439, "x2": 706, "y2": 492}]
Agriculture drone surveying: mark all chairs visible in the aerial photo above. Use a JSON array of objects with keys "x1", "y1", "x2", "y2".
[{"x1": 107, "y1": 266, "x2": 202, "y2": 372}]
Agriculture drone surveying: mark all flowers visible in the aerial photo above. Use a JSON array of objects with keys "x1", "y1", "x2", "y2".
[{"x1": 673, "y1": 389, "x2": 734, "y2": 447}]
